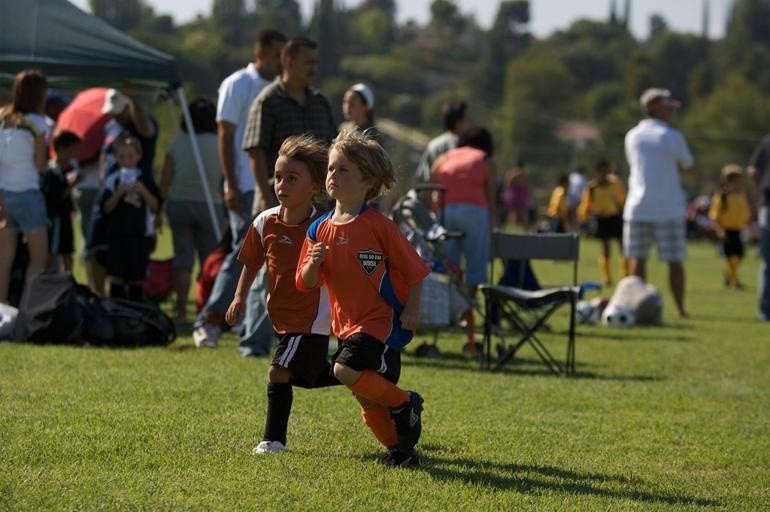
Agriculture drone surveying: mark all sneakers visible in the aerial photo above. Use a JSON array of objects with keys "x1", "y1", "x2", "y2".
[
  {"x1": 390, "y1": 392, "x2": 424, "y2": 448},
  {"x1": 380, "y1": 449, "x2": 419, "y2": 467}
]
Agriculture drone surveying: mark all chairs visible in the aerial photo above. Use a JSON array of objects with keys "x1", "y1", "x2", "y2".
[{"x1": 480, "y1": 231, "x2": 583, "y2": 376}]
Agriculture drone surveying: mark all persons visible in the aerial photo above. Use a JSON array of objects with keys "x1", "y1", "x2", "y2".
[
  {"x1": 297, "y1": 125, "x2": 432, "y2": 470},
  {"x1": 413, "y1": 87, "x2": 770, "y2": 361},
  {"x1": 1, "y1": 31, "x2": 392, "y2": 359},
  {"x1": 224, "y1": 133, "x2": 357, "y2": 463}
]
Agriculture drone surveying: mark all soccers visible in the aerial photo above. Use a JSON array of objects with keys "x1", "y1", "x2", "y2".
[{"x1": 602, "y1": 304, "x2": 638, "y2": 328}]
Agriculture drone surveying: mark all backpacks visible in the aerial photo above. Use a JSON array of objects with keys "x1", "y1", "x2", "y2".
[{"x1": 19, "y1": 271, "x2": 176, "y2": 347}]
